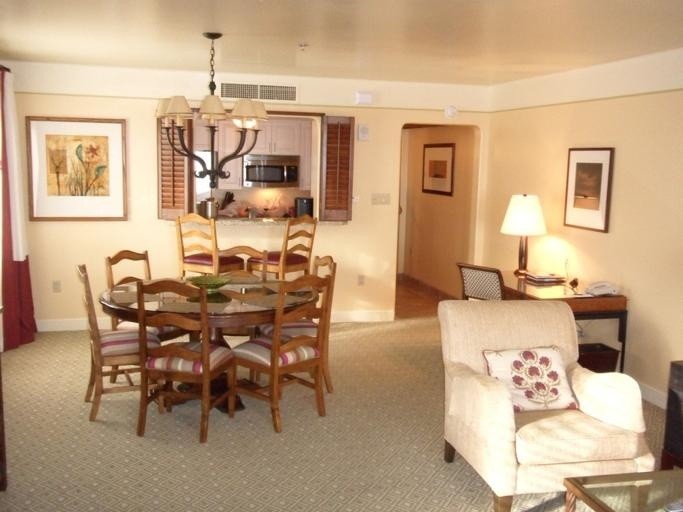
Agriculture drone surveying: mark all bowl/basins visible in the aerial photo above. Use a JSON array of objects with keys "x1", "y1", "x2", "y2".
[
  {"x1": 187, "y1": 275, "x2": 231, "y2": 290},
  {"x1": 187, "y1": 294, "x2": 231, "y2": 306}
]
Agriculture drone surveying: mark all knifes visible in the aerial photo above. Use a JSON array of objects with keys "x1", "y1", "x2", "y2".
[{"x1": 224, "y1": 192, "x2": 234, "y2": 201}]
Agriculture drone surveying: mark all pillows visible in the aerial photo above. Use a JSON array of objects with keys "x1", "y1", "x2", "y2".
[{"x1": 481, "y1": 343, "x2": 580, "y2": 413}]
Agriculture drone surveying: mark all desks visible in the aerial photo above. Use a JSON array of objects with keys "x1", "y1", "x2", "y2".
[{"x1": 498, "y1": 269, "x2": 628, "y2": 373}]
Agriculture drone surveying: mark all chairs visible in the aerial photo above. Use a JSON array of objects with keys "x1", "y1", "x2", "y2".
[
  {"x1": 455, "y1": 261, "x2": 507, "y2": 301},
  {"x1": 436, "y1": 297, "x2": 658, "y2": 512},
  {"x1": 78, "y1": 212, "x2": 336, "y2": 445}
]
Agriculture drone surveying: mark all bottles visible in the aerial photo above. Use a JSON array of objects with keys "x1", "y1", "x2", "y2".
[
  {"x1": 295, "y1": 195, "x2": 313, "y2": 218},
  {"x1": 200, "y1": 200, "x2": 220, "y2": 218}
]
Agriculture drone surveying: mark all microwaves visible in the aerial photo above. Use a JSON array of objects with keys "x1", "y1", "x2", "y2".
[{"x1": 242, "y1": 153, "x2": 299, "y2": 187}]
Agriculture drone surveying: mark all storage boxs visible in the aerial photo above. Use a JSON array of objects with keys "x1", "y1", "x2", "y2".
[{"x1": 579, "y1": 340, "x2": 623, "y2": 373}]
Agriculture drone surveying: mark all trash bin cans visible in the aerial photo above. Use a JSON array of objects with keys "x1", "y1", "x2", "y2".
[{"x1": 577, "y1": 343, "x2": 620, "y2": 373}]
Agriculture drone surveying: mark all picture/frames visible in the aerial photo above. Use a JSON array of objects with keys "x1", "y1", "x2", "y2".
[
  {"x1": 23, "y1": 114, "x2": 131, "y2": 224},
  {"x1": 421, "y1": 142, "x2": 455, "y2": 197},
  {"x1": 561, "y1": 147, "x2": 616, "y2": 235}
]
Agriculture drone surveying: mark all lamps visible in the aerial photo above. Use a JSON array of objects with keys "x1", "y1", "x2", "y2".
[
  {"x1": 152, "y1": 29, "x2": 269, "y2": 190},
  {"x1": 498, "y1": 192, "x2": 548, "y2": 278}
]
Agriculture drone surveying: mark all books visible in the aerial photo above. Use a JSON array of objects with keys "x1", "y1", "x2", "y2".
[{"x1": 527, "y1": 273, "x2": 566, "y2": 286}]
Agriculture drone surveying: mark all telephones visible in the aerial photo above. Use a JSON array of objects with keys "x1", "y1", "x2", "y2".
[{"x1": 585, "y1": 282, "x2": 617, "y2": 297}]
